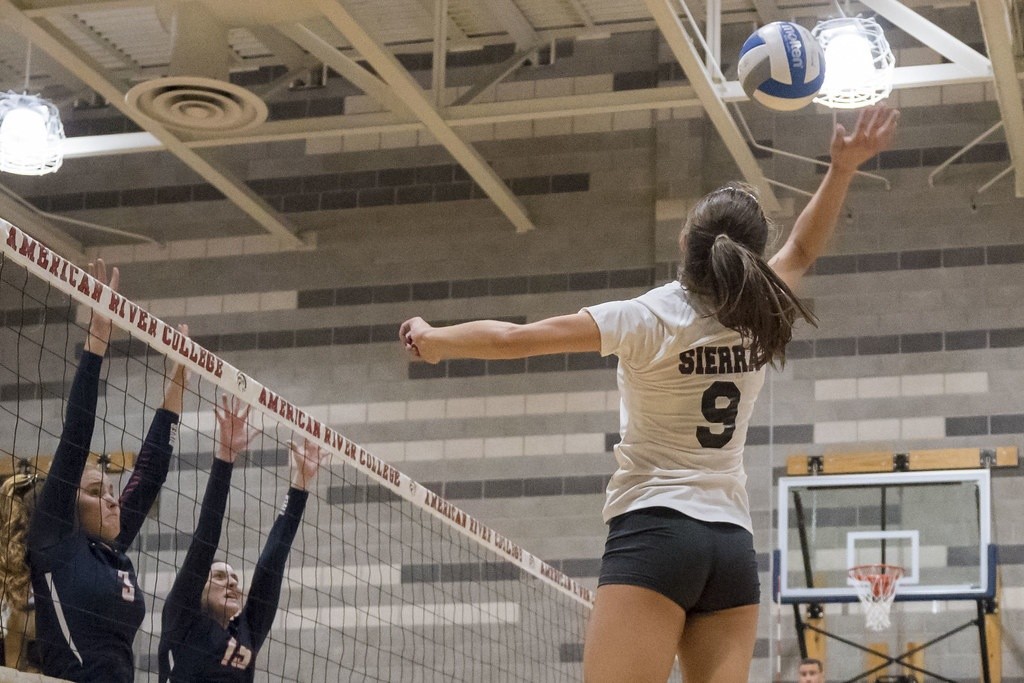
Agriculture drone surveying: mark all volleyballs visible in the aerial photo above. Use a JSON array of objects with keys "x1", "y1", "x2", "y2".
[{"x1": 737, "y1": 21, "x2": 829, "y2": 113}]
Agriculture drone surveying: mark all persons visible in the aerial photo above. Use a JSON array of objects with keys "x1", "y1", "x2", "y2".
[
  {"x1": 797, "y1": 657, "x2": 824, "y2": 683},
  {"x1": 158, "y1": 393, "x2": 331, "y2": 683},
  {"x1": 1, "y1": 256, "x2": 190, "y2": 683},
  {"x1": 399, "y1": 100, "x2": 900, "y2": 683}
]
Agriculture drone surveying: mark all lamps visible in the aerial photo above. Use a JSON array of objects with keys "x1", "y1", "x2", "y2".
[
  {"x1": 811, "y1": 0, "x2": 897, "y2": 111},
  {"x1": 0, "y1": 36, "x2": 67, "y2": 177}
]
